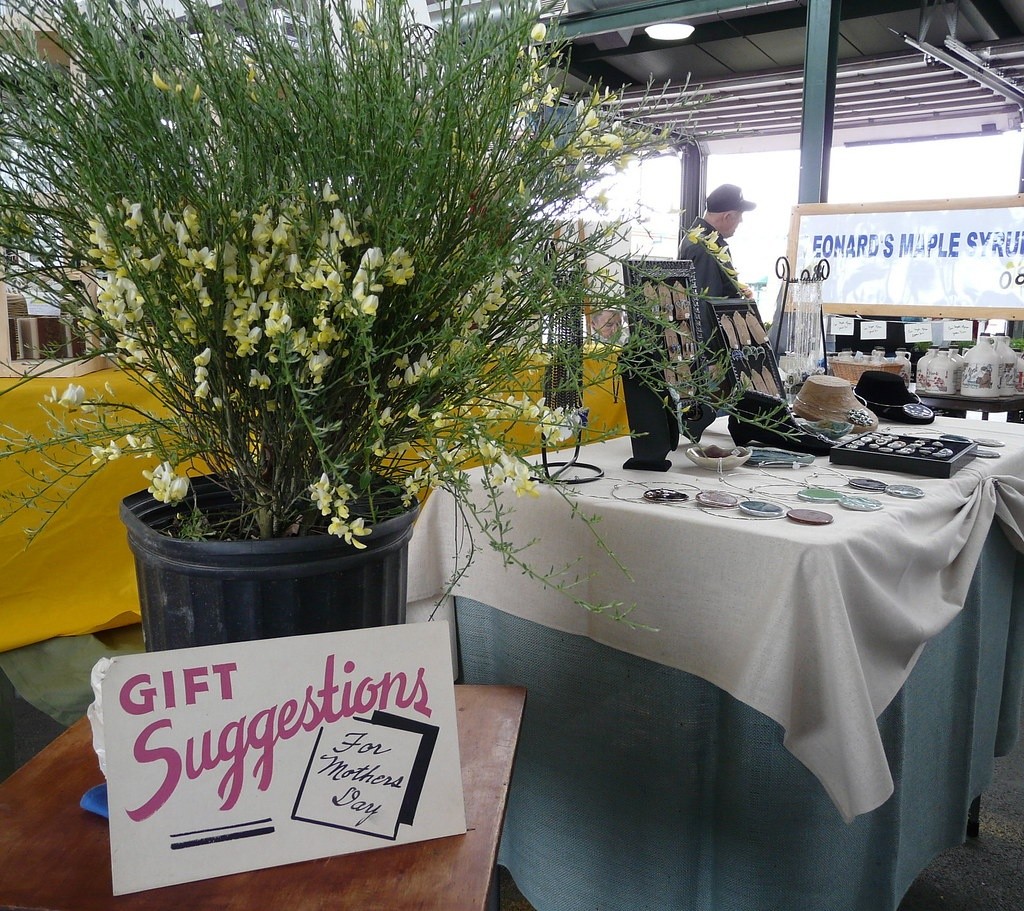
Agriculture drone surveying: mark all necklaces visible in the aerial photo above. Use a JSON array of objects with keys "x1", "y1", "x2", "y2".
[
  {"x1": 795, "y1": 393, "x2": 873, "y2": 428},
  {"x1": 565, "y1": 406, "x2": 1007, "y2": 526},
  {"x1": 851, "y1": 391, "x2": 934, "y2": 420}
]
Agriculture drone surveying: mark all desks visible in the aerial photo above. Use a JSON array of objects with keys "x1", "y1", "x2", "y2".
[
  {"x1": 907, "y1": 383, "x2": 1024, "y2": 423},
  {"x1": 401, "y1": 415, "x2": 1024, "y2": 911},
  {"x1": 0, "y1": 681, "x2": 528, "y2": 911}
]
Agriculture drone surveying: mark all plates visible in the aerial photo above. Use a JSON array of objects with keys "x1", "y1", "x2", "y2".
[{"x1": 685, "y1": 444, "x2": 753, "y2": 471}]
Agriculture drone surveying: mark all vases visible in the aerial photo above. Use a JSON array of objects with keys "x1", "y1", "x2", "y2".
[{"x1": 118, "y1": 472, "x2": 422, "y2": 653}]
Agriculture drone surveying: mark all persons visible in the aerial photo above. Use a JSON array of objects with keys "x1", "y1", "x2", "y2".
[{"x1": 676, "y1": 184, "x2": 757, "y2": 447}]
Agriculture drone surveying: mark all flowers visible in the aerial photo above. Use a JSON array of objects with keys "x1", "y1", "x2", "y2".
[{"x1": 0, "y1": 0, "x2": 837, "y2": 635}]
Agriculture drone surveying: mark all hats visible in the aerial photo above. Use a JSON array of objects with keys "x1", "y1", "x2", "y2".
[{"x1": 706, "y1": 184, "x2": 757, "y2": 213}]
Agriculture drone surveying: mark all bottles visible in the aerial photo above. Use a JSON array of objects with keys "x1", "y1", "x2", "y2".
[
  {"x1": 915, "y1": 333, "x2": 1024, "y2": 398},
  {"x1": 838, "y1": 345, "x2": 912, "y2": 389}
]
